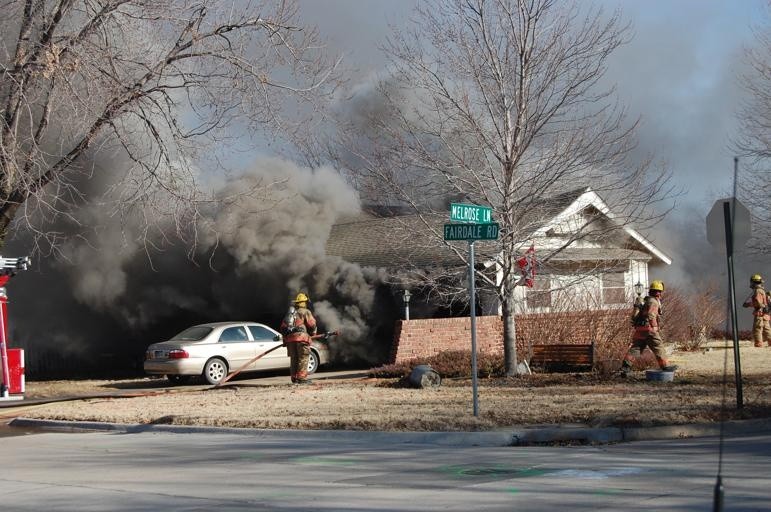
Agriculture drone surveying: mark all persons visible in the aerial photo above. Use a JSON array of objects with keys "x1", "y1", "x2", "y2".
[
  {"x1": 743, "y1": 274, "x2": 771, "y2": 347},
  {"x1": 280, "y1": 292, "x2": 318, "y2": 385},
  {"x1": 622, "y1": 280, "x2": 679, "y2": 379}
]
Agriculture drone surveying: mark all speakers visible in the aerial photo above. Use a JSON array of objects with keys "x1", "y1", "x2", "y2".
[
  {"x1": 621, "y1": 366, "x2": 629, "y2": 378},
  {"x1": 662, "y1": 365, "x2": 679, "y2": 372}
]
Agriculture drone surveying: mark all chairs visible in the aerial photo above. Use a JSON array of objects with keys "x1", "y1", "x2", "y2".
[{"x1": 292, "y1": 378, "x2": 312, "y2": 384}]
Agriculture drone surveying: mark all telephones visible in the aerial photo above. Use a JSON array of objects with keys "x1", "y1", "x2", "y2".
[{"x1": 444, "y1": 203, "x2": 499, "y2": 241}]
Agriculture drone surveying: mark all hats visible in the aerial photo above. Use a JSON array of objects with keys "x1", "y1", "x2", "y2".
[{"x1": 143, "y1": 320, "x2": 331, "y2": 385}]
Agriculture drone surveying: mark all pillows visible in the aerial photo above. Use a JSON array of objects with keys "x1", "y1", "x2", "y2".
[
  {"x1": 750, "y1": 274, "x2": 762, "y2": 283},
  {"x1": 650, "y1": 280, "x2": 665, "y2": 291},
  {"x1": 291, "y1": 292, "x2": 310, "y2": 303}
]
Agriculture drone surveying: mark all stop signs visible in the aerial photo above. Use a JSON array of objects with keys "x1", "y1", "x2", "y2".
[{"x1": 1, "y1": 255, "x2": 31, "y2": 402}]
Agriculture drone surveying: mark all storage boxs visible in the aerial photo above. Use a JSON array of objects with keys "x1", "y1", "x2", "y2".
[{"x1": 529, "y1": 341, "x2": 594, "y2": 371}]
